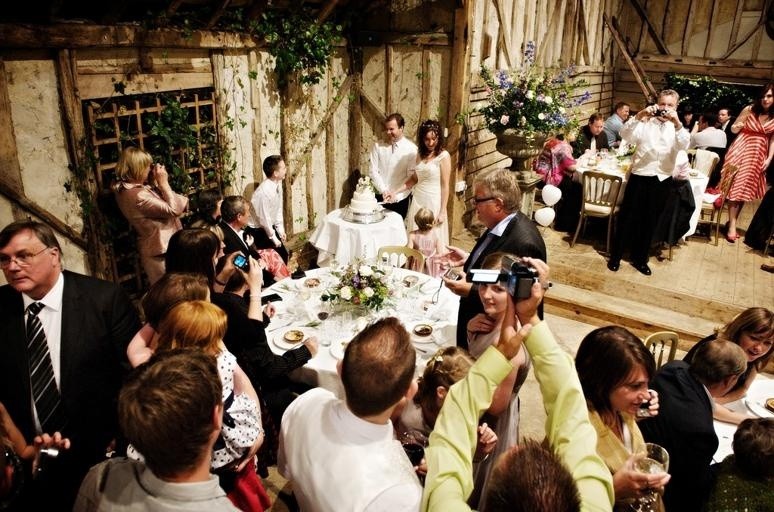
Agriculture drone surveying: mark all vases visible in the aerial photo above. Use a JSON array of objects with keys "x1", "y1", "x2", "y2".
[{"x1": 490, "y1": 127, "x2": 552, "y2": 220}]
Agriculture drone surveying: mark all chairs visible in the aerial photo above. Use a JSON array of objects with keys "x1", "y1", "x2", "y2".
[
  {"x1": 663, "y1": 177, "x2": 697, "y2": 260},
  {"x1": 643, "y1": 330, "x2": 681, "y2": 373},
  {"x1": 687, "y1": 150, "x2": 720, "y2": 174},
  {"x1": 700, "y1": 163, "x2": 741, "y2": 246},
  {"x1": 570, "y1": 170, "x2": 622, "y2": 258},
  {"x1": 375, "y1": 246, "x2": 424, "y2": 273}
]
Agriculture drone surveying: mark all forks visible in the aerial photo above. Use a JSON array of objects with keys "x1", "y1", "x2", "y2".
[{"x1": 269, "y1": 321, "x2": 294, "y2": 332}]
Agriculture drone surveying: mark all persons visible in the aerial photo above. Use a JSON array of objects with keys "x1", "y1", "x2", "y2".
[
  {"x1": 72, "y1": 353, "x2": 244, "y2": 512},
  {"x1": 370, "y1": 113, "x2": 419, "y2": 221},
  {"x1": 714, "y1": 107, "x2": 738, "y2": 152},
  {"x1": 249, "y1": 155, "x2": 289, "y2": 266},
  {"x1": 533, "y1": 125, "x2": 583, "y2": 231},
  {"x1": 216, "y1": 254, "x2": 320, "y2": 398},
  {"x1": 433, "y1": 166, "x2": 546, "y2": 351},
  {"x1": 681, "y1": 107, "x2": 695, "y2": 132},
  {"x1": 604, "y1": 102, "x2": 633, "y2": 147},
  {"x1": 671, "y1": 150, "x2": 690, "y2": 182},
  {"x1": 467, "y1": 251, "x2": 531, "y2": 512},
  {"x1": 0, "y1": 221, "x2": 143, "y2": 512},
  {"x1": 126, "y1": 301, "x2": 265, "y2": 473},
  {"x1": 570, "y1": 112, "x2": 610, "y2": 160},
  {"x1": 688, "y1": 113, "x2": 727, "y2": 150},
  {"x1": 193, "y1": 219, "x2": 226, "y2": 259},
  {"x1": 675, "y1": 418, "x2": 774, "y2": 512},
  {"x1": 111, "y1": 145, "x2": 190, "y2": 283},
  {"x1": 277, "y1": 318, "x2": 423, "y2": 512},
  {"x1": 393, "y1": 346, "x2": 498, "y2": 487},
  {"x1": 574, "y1": 325, "x2": 671, "y2": 512},
  {"x1": 1, "y1": 403, "x2": 70, "y2": 512},
  {"x1": 721, "y1": 82, "x2": 774, "y2": 243},
  {"x1": 218, "y1": 196, "x2": 278, "y2": 288},
  {"x1": 419, "y1": 259, "x2": 615, "y2": 512},
  {"x1": 637, "y1": 339, "x2": 746, "y2": 512},
  {"x1": 134, "y1": 228, "x2": 273, "y2": 391},
  {"x1": 403, "y1": 119, "x2": 451, "y2": 253},
  {"x1": 607, "y1": 89, "x2": 690, "y2": 275},
  {"x1": 139, "y1": 273, "x2": 272, "y2": 512},
  {"x1": 682, "y1": 306, "x2": 774, "y2": 425},
  {"x1": 405, "y1": 207, "x2": 449, "y2": 280}
]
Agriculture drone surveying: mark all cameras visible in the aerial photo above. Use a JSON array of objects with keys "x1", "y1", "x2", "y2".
[
  {"x1": 469, "y1": 260, "x2": 553, "y2": 301},
  {"x1": 149, "y1": 163, "x2": 162, "y2": 175},
  {"x1": 233, "y1": 254, "x2": 250, "y2": 272},
  {"x1": 654, "y1": 109, "x2": 667, "y2": 116},
  {"x1": 636, "y1": 391, "x2": 653, "y2": 419}
]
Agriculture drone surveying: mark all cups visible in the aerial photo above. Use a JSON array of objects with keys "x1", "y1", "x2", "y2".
[
  {"x1": 600, "y1": 148, "x2": 609, "y2": 163},
  {"x1": 320, "y1": 320, "x2": 333, "y2": 346},
  {"x1": 383, "y1": 261, "x2": 393, "y2": 284},
  {"x1": 626, "y1": 442, "x2": 671, "y2": 512},
  {"x1": 341, "y1": 310, "x2": 353, "y2": 332},
  {"x1": 329, "y1": 255, "x2": 339, "y2": 273},
  {"x1": 621, "y1": 158, "x2": 631, "y2": 177},
  {"x1": 394, "y1": 271, "x2": 403, "y2": 289},
  {"x1": 315, "y1": 305, "x2": 329, "y2": 333},
  {"x1": 402, "y1": 430, "x2": 424, "y2": 467}
]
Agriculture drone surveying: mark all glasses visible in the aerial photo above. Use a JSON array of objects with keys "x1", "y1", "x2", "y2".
[
  {"x1": 0, "y1": 244, "x2": 51, "y2": 267},
  {"x1": 474, "y1": 197, "x2": 495, "y2": 206}
]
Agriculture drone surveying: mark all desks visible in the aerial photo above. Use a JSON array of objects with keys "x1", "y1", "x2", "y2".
[{"x1": 305, "y1": 206, "x2": 411, "y2": 268}]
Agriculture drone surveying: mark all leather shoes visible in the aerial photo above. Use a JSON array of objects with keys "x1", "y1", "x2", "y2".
[
  {"x1": 607, "y1": 257, "x2": 621, "y2": 271},
  {"x1": 725, "y1": 232, "x2": 737, "y2": 242},
  {"x1": 632, "y1": 259, "x2": 651, "y2": 275}
]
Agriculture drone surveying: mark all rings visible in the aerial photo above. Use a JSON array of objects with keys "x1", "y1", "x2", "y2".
[
  {"x1": 652, "y1": 106, "x2": 657, "y2": 110},
  {"x1": 638, "y1": 482, "x2": 655, "y2": 495}
]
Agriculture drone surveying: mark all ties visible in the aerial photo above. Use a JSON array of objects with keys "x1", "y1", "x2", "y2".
[
  {"x1": 660, "y1": 123, "x2": 666, "y2": 133},
  {"x1": 467, "y1": 232, "x2": 494, "y2": 273},
  {"x1": 23, "y1": 302, "x2": 64, "y2": 431},
  {"x1": 277, "y1": 186, "x2": 280, "y2": 193},
  {"x1": 590, "y1": 135, "x2": 597, "y2": 155},
  {"x1": 391, "y1": 144, "x2": 396, "y2": 155}
]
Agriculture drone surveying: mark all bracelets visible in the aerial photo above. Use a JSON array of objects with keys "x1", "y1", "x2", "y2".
[{"x1": 215, "y1": 278, "x2": 226, "y2": 288}]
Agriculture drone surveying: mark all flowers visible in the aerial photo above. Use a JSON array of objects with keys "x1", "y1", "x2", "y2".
[{"x1": 477, "y1": 40, "x2": 591, "y2": 137}]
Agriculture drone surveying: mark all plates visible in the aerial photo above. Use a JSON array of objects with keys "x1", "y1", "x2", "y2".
[
  {"x1": 412, "y1": 323, "x2": 433, "y2": 337},
  {"x1": 295, "y1": 276, "x2": 323, "y2": 291},
  {"x1": 410, "y1": 334, "x2": 435, "y2": 344},
  {"x1": 303, "y1": 277, "x2": 321, "y2": 288},
  {"x1": 273, "y1": 332, "x2": 309, "y2": 350},
  {"x1": 284, "y1": 330, "x2": 304, "y2": 342},
  {"x1": 745, "y1": 379, "x2": 774, "y2": 418},
  {"x1": 765, "y1": 397, "x2": 774, "y2": 411},
  {"x1": 329, "y1": 340, "x2": 350, "y2": 360},
  {"x1": 402, "y1": 274, "x2": 420, "y2": 289}
]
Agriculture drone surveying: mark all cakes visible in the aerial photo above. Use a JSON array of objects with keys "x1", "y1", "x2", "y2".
[{"x1": 346, "y1": 178, "x2": 384, "y2": 222}]
